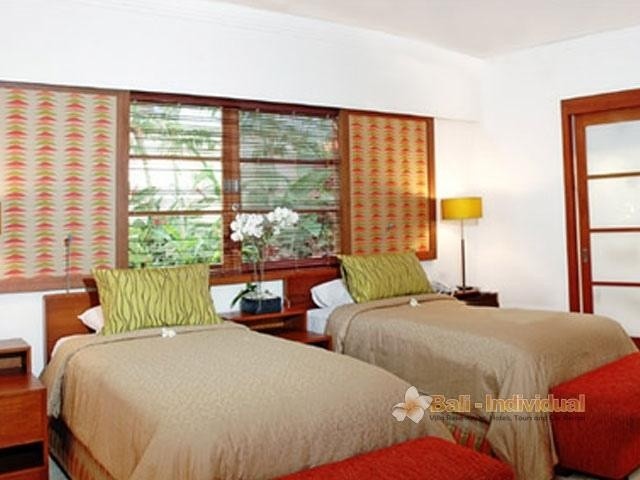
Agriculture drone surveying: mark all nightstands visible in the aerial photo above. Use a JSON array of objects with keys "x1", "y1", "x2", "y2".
[
  {"x1": 452, "y1": 290, "x2": 500, "y2": 307},
  {"x1": 0, "y1": 338, "x2": 49, "y2": 480},
  {"x1": 220, "y1": 303, "x2": 331, "y2": 352}
]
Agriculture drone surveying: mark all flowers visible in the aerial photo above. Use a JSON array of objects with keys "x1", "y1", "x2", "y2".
[{"x1": 230, "y1": 207, "x2": 300, "y2": 296}]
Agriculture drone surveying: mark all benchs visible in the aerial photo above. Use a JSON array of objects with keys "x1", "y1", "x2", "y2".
[
  {"x1": 271, "y1": 434, "x2": 516, "y2": 480},
  {"x1": 548, "y1": 353, "x2": 640, "y2": 479}
]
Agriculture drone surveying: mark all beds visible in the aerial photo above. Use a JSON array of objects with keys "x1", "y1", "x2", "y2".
[
  {"x1": 284, "y1": 267, "x2": 640, "y2": 479},
  {"x1": 44, "y1": 293, "x2": 457, "y2": 479}
]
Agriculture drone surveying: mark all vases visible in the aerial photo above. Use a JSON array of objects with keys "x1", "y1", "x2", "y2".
[{"x1": 241, "y1": 294, "x2": 280, "y2": 313}]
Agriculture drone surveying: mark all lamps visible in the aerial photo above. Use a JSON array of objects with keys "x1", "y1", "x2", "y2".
[{"x1": 441, "y1": 197, "x2": 482, "y2": 298}]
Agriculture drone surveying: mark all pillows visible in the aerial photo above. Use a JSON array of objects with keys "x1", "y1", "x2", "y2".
[
  {"x1": 338, "y1": 250, "x2": 434, "y2": 303},
  {"x1": 94, "y1": 264, "x2": 222, "y2": 336}
]
